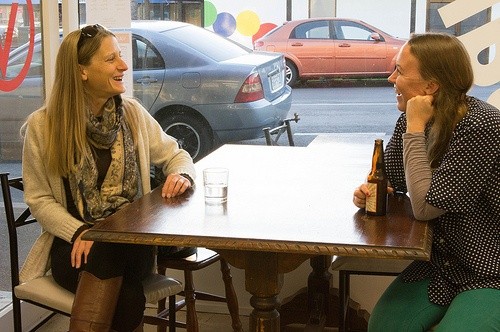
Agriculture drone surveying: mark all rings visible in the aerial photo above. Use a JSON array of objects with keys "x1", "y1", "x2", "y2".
[{"x1": 355, "y1": 196, "x2": 357, "y2": 201}]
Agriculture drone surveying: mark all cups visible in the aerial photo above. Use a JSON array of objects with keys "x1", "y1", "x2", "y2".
[{"x1": 203, "y1": 168, "x2": 228, "y2": 203}]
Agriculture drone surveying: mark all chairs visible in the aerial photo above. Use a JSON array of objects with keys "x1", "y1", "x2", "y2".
[
  {"x1": 143, "y1": 246, "x2": 244, "y2": 332},
  {"x1": 0, "y1": 172, "x2": 183, "y2": 332},
  {"x1": 331, "y1": 256, "x2": 413, "y2": 332}
]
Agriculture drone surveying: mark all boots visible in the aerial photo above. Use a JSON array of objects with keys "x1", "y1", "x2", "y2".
[
  {"x1": 68, "y1": 270, "x2": 122, "y2": 332},
  {"x1": 116, "y1": 296, "x2": 146, "y2": 332}
]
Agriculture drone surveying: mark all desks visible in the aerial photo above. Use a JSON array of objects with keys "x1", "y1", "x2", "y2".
[{"x1": 81, "y1": 143, "x2": 433, "y2": 332}]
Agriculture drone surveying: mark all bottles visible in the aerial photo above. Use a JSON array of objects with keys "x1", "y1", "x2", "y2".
[{"x1": 365, "y1": 139, "x2": 387, "y2": 216}]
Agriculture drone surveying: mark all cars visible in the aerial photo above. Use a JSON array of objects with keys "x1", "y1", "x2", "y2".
[
  {"x1": 254, "y1": 17, "x2": 409, "y2": 87},
  {"x1": 0, "y1": 20, "x2": 41, "y2": 43},
  {"x1": 0, "y1": 19, "x2": 296, "y2": 164}
]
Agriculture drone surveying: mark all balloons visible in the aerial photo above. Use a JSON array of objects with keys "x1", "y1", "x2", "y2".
[{"x1": 204, "y1": 0, "x2": 278, "y2": 47}]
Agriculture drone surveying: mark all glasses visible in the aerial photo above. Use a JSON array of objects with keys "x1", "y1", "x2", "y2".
[{"x1": 77, "y1": 22, "x2": 102, "y2": 54}]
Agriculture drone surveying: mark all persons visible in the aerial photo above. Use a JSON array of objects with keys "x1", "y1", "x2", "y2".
[
  {"x1": 353, "y1": 34, "x2": 500, "y2": 332},
  {"x1": 19, "y1": 24, "x2": 196, "y2": 332}
]
